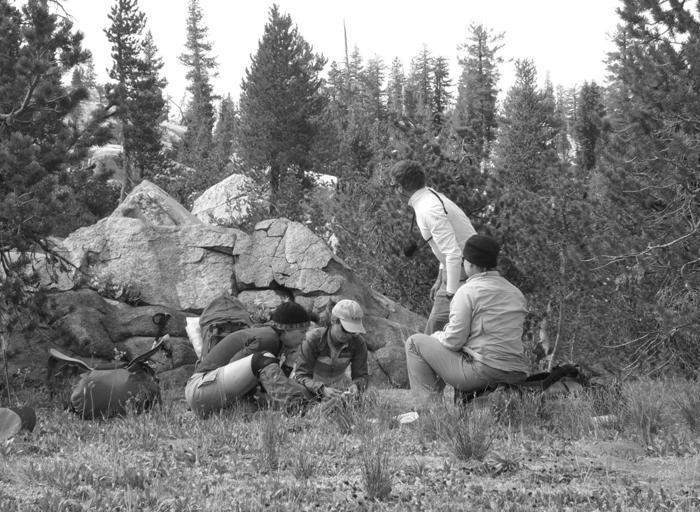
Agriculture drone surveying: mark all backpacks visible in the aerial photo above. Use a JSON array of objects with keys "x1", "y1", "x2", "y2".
[
  {"x1": 44, "y1": 332, "x2": 171, "y2": 419},
  {"x1": 525, "y1": 363, "x2": 586, "y2": 396},
  {"x1": 194, "y1": 295, "x2": 252, "y2": 365}
]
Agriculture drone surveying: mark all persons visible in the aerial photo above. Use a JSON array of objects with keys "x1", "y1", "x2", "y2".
[
  {"x1": 402, "y1": 233, "x2": 530, "y2": 414},
  {"x1": 387, "y1": 156, "x2": 481, "y2": 394},
  {"x1": 289, "y1": 297, "x2": 374, "y2": 414},
  {"x1": 185, "y1": 298, "x2": 324, "y2": 432}
]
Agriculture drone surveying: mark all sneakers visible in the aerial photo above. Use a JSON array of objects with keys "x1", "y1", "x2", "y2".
[{"x1": 302, "y1": 397, "x2": 337, "y2": 423}]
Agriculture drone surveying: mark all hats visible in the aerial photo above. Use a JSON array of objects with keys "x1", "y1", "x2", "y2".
[
  {"x1": 332, "y1": 299, "x2": 366, "y2": 335},
  {"x1": 272, "y1": 301, "x2": 320, "y2": 324},
  {"x1": 463, "y1": 235, "x2": 501, "y2": 267}
]
